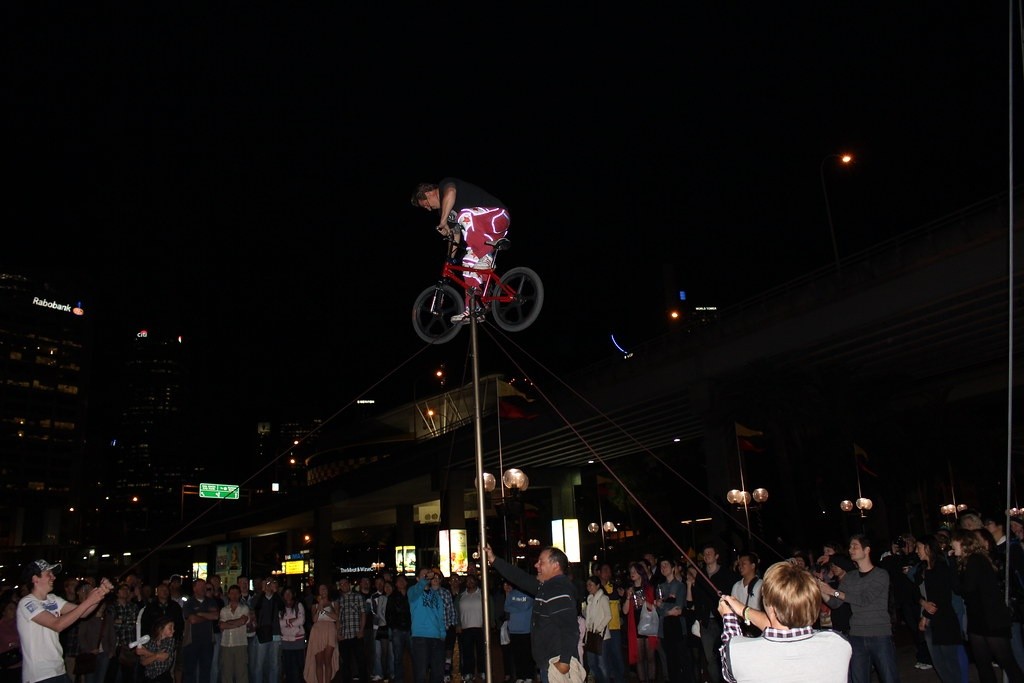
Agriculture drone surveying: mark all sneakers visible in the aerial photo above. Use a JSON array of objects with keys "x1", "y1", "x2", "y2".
[
  {"x1": 450, "y1": 307, "x2": 486, "y2": 323},
  {"x1": 473, "y1": 254, "x2": 497, "y2": 269}
]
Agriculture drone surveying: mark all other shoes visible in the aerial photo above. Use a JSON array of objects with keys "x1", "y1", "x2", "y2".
[
  {"x1": 462, "y1": 673, "x2": 473, "y2": 683},
  {"x1": 504, "y1": 675, "x2": 510, "y2": 681},
  {"x1": 524, "y1": 678, "x2": 532, "y2": 683},
  {"x1": 444, "y1": 675, "x2": 450, "y2": 683},
  {"x1": 383, "y1": 679, "x2": 392, "y2": 683},
  {"x1": 481, "y1": 673, "x2": 485, "y2": 680},
  {"x1": 389, "y1": 673, "x2": 395, "y2": 679},
  {"x1": 370, "y1": 674, "x2": 383, "y2": 681},
  {"x1": 515, "y1": 679, "x2": 523, "y2": 683},
  {"x1": 914, "y1": 662, "x2": 933, "y2": 669},
  {"x1": 991, "y1": 662, "x2": 999, "y2": 667},
  {"x1": 353, "y1": 678, "x2": 359, "y2": 680},
  {"x1": 450, "y1": 675, "x2": 453, "y2": 680}
]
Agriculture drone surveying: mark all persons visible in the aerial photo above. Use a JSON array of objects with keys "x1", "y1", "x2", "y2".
[
  {"x1": 411, "y1": 176, "x2": 511, "y2": 325},
  {"x1": 1, "y1": 513, "x2": 1024, "y2": 682}
]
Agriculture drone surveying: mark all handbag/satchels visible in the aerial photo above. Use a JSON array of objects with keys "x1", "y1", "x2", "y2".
[
  {"x1": 72, "y1": 653, "x2": 97, "y2": 675},
  {"x1": 586, "y1": 631, "x2": 603, "y2": 656},
  {"x1": 119, "y1": 646, "x2": 136, "y2": 669},
  {"x1": 255, "y1": 628, "x2": 273, "y2": 643},
  {"x1": 636, "y1": 601, "x2": 660, "y2": 636}
]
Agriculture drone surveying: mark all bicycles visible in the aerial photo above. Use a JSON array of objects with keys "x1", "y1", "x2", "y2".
[{"x1": 412, "y1": 220, "x2": 545, "y2": 347}]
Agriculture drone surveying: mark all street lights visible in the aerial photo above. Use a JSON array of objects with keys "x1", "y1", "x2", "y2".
[
  {"x1": 727, "y1": 488, "x2": 769, "y2": 550},
  {"x1": 473, "y1": 468, "x2": 532, "y2": 561},
  {"x1": 587, "y1": 522, "x2": 618, "y2": 566},
  {"x1": 841, "y1": 497, "x2": 872, "y2": 534}
]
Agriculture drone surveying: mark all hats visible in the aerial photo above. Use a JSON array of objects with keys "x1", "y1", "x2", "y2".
[{"x1": 23, "y1": 559, "x2": 62, "y2": 578}]
